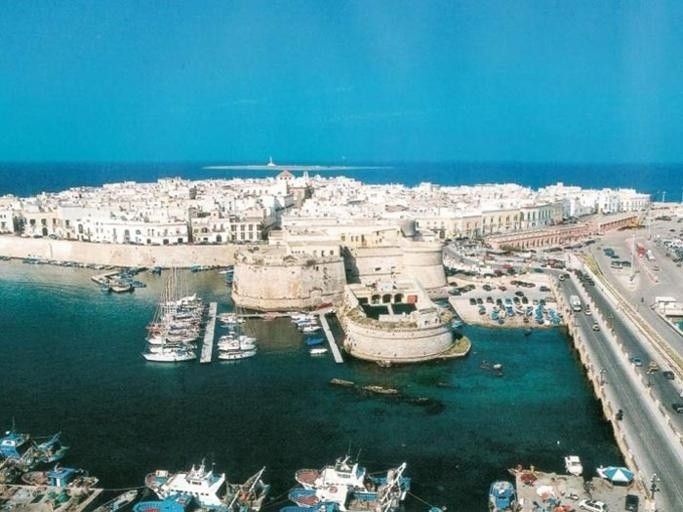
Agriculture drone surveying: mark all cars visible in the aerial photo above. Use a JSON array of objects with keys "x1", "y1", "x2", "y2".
[
  {"x1": 625, "y1": 494, "x2": 637, "y2": 512},
  {"x1": 448, "y1": 278, "x2": 563, "y2": 327},
  {"x1": 566, "y1": 237, "x2": 682, "y2": 422}
]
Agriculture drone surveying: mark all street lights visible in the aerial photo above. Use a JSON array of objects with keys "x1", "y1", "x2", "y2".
[
  {"x1": 599, "y1": 366, "x2": 607, "y2": 385},
  {"x1": 649, "y1": 473, "x2": 661, "y2": 498}
]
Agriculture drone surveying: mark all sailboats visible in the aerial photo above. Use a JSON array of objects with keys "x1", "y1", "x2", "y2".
[{"x1": 138, "y1": 261, "x2": 207, "y2": 365}]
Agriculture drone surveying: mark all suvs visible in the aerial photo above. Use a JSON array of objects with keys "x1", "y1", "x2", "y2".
[{"x1": 579, "y1": 500, "x2": 608, "y2": 512}]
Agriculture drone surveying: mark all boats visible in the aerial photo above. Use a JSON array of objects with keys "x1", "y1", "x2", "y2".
[
  {"x1": 92, "y1": 489, "x2": 139, "y2": 512},
  {"x1": 1, "y1": 419, "x2": 103, "y2": 512},
  {"x1": 488, "y1": 479, "x2": 517, "y2": 512},
  {"x1": 133, "y1": 456, "x2": 272, "y2": 512},
  {"x1": 566, "y1": 455, "x2": 583, "y2": 477},
  {"x1": 90, "y1": 268, "x2": 142, "y2": 293},
  {"x1": 216, "y1": 311, "x2": 331, "y2": 363},
  {"x1": 279, "y1": 440, "x2": 412, "y2": 512}
]
